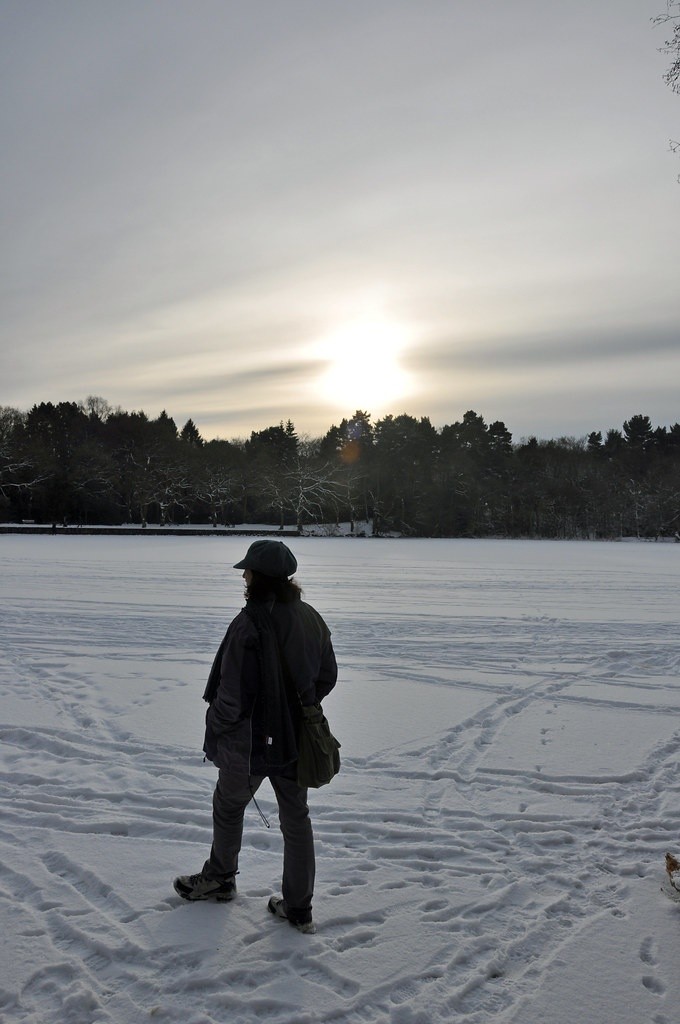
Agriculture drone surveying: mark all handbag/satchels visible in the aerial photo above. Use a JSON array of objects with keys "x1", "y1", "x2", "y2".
[{"x1": 298, "y1": 704, "x2": 341, "y2": 789}]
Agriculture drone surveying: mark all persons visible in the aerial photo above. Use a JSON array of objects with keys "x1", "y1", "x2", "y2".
[{"x1": 173, "y1": 539, "x2": 342, "y2": 934}]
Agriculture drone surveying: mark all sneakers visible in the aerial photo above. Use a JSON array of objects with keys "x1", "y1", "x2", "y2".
[
  {"x1": 173, "y1": 857, "x2": 240, "y2": 903},
  {"x1": 267, "y1": 895, "x2": 316, "y2": 933}
]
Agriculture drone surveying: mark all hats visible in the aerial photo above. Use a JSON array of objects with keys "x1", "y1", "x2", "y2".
[{"x1": 233, "y1": 539, "x2": 297, "y2": 576}]
